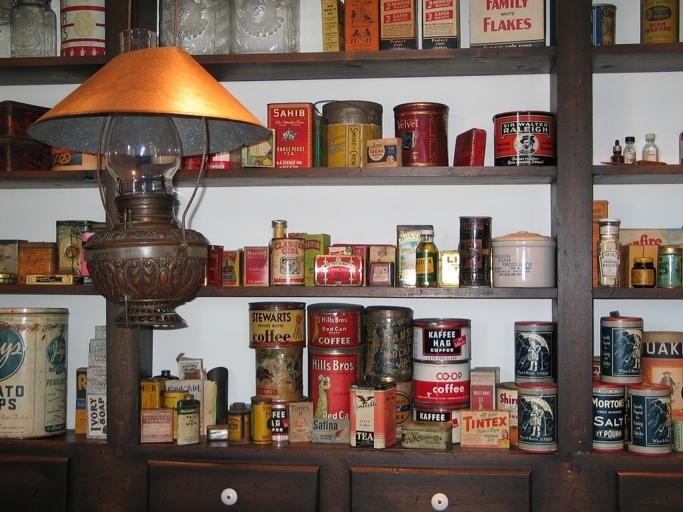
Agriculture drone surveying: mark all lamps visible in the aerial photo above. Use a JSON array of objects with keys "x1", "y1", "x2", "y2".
[{"x1": 29, "y1": 1, "x2": 274, "y2": 327}]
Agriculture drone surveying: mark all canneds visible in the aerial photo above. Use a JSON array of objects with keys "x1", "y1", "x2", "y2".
[
  {"x1": 412, "y1": 405, "x2": 462, "y2": 445},
  {"x1": 248, "y1": 301, "x2": 304, "y2": 348},
  {"x1": 268, "y1": 237, "x2": 306, "y2": 286},
  {"x1": 364, "y1": 306, "x2": 414, "y2": 379},
  {"x1": 591, "y1": 4, "x2": 616, "y2": 48},
  {"x1": 492, "y1": 110, "x2": 556, "y2": 166},
  {"x1": 393, "y1": 103, "x2": 449, "y2": 166},
  {"x1": 412, "y1": 319, "x2": 471, "y2": 364},
  {"x1": 413, "y1": 363, "x2": 470, "y2": 405},
  {"x1": 256, "y1": 348, "x2": 302, "y2": 400},
  {"x1": 308, "y1": 348, "x2": 364, "y2": 444},
  {"x1": 363, "y1": 378, "x2": 412, "y2": 439},
  {"x1": 269, "y1": 397, "x2": 308, "y2": 444},
  {"x1": 309, "y1": 305, "x2": 363, "y2": 349}
]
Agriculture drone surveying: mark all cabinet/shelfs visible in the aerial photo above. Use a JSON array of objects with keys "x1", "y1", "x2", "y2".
[
  {"x1": 0, "y1": 1, "x2": 683, "y2": 455},
  {"x1": 0, "y1": 440, "x2": 682, "y2": 512}
]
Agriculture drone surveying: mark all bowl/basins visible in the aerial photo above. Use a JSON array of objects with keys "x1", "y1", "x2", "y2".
[{"x1": 493, "y1": 232, "x2": 557, "y2": 288}]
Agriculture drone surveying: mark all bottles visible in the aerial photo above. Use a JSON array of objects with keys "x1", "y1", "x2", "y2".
[
  {"x1": 609, "y1": 139, "x2": 622, "y2": 165},
  {"x1": 598, "y1": 218, "x2": 622, "y2": 288},
  {"x1": 415, "y1": 230, "x2": 439, "y2": 287},
  {"x1": 630, "y1": 255, "x2": 656, "y2": 288},
  {"x1": 641, "y1": 134, "x2": 658, "y2": 161},
  {"x1": 623, "y1": 136, "x2": 634, "y2": 165}
]
